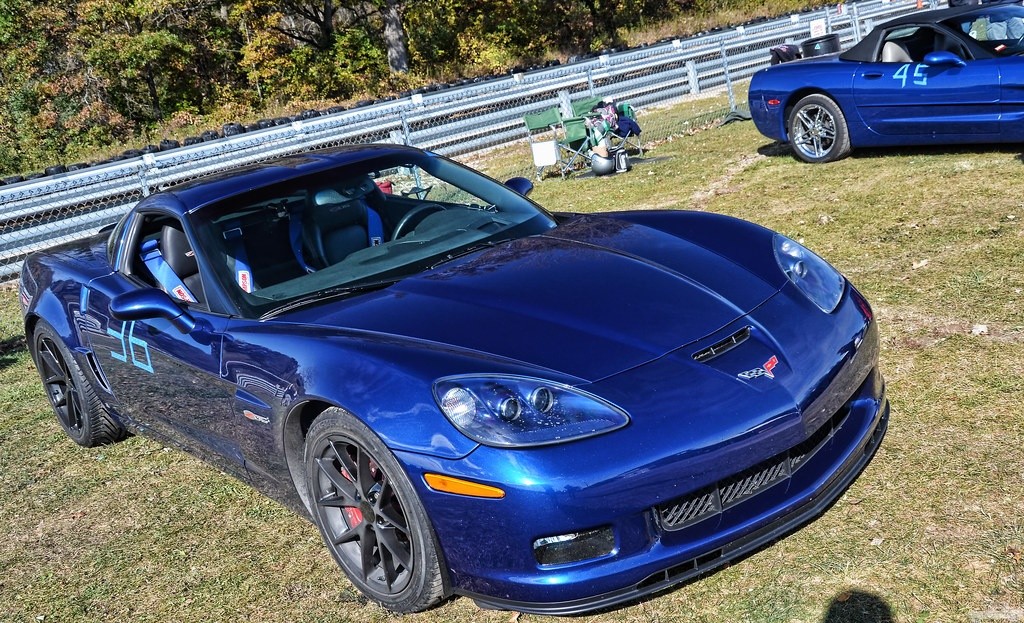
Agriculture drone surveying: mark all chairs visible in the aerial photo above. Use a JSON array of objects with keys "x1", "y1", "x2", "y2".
[
  {"x1": 160, "y1": 215, "x2": 263, "y2": 304},
  {"x1": 523, "y1": 94, "x2": 643, "y2": 183},
  {"x1": 301, "y1": 166, "x2": 402, "y2": 271},
  {"x1": 934, "y1": 25, "x2": 982, "y2": 60}
]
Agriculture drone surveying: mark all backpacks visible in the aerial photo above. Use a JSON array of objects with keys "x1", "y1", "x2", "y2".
[{"x1": 616, "y1": 148, "x2": 632, "y2": 173}]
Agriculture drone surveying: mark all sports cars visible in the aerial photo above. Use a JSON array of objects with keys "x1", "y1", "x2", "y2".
[{"x1": 19, "y1": 143, "x2": 890, "y2": 615}]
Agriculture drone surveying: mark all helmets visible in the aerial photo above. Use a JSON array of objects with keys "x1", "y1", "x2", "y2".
[{"x1": 591, "y1": 153, "x2": 615, "y2": 175}]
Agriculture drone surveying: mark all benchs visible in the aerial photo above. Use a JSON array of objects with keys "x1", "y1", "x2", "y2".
[
  {"x1": 881, "y1": 28, "x2": 973, "y2": 63},
  {"x1": 135, "y1": 200, "x2": 309, "y2": 290}
]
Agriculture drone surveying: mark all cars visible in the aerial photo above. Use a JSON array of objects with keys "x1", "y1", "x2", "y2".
[{"x1": 748, "y1": 4, "x2": 1024, "y2": 164}]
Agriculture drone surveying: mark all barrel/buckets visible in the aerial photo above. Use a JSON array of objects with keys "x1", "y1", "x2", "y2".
[{"x1": 800, "y1": 33, "x2": 841, "y2": 57}]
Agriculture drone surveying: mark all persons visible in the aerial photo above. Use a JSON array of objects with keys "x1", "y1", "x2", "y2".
[
  {"x1": 948, "y1": 0, "x2": 978, "y2": 35},
  {"x1": 982, "y1": 0, "x2": 1024, "y2": 40}
]
